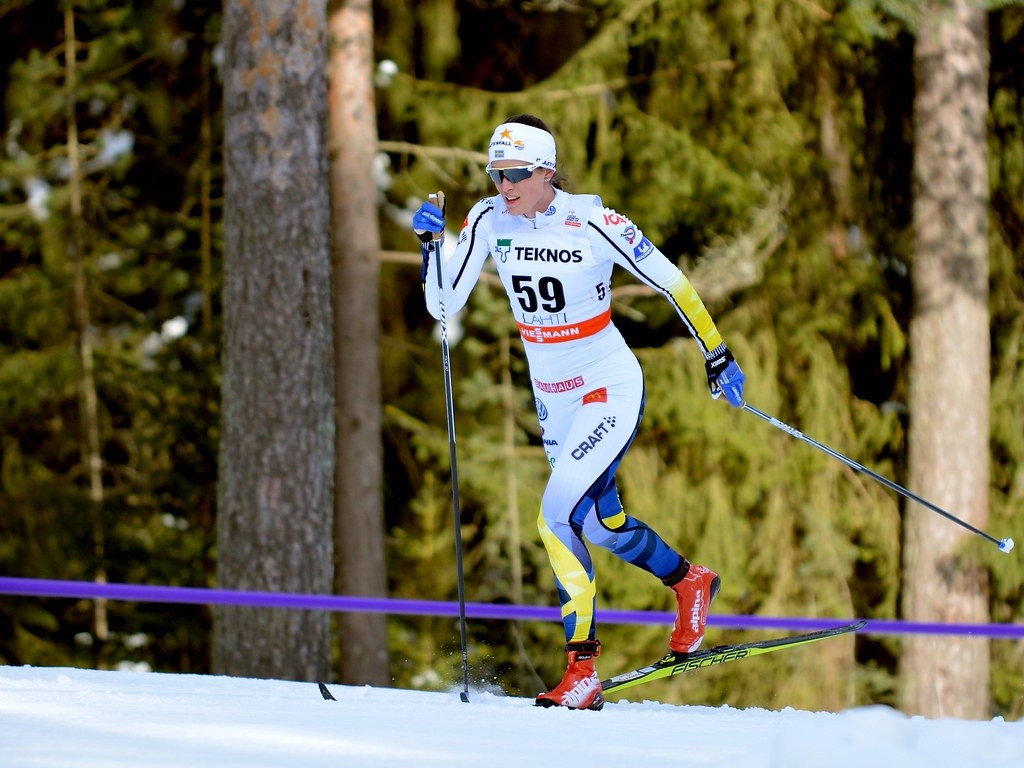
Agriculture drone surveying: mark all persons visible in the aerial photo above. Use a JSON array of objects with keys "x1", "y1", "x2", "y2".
[{"x1": 411, "y1": 113, "x2": 747, "y2": 712}]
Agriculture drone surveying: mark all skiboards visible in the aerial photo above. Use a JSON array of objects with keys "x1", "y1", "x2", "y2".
[{"x1": 317, "y1": 619, "x2": 870, "y2": 711}]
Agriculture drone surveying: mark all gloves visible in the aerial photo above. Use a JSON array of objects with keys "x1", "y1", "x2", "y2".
[
  {"x1": 705, "y1": 348, "x2": 745, "y2": 408},
  {"x1": 413, "y1": 202, "x2": 447, "y2": 251}
]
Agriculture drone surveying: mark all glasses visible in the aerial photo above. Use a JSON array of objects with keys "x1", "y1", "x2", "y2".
[{"x1": 485, "y1": 153, "x2": 554, "y2": 185}]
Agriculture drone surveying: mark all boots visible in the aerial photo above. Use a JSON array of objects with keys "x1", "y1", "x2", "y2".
[
  {"x1": 660, "y1": 554, "x2": 721, "y2": 653},
  {"x1": 535, "y1": 640, "x2": 605, "y2": 712}
]
